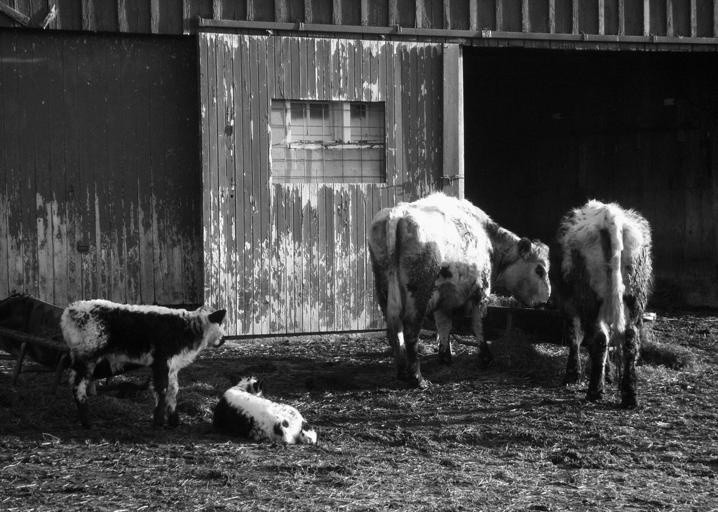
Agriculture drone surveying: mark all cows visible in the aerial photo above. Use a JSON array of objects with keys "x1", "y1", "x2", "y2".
[
  {"x1": 557, "y1": 197, "x2": 657, "y2": 411},
  {"x1": 58, "y1": 298, "x2": 228, "y2": 429},
  {"x1": 213, "y1": 371, "x2": 318, "y2": 446},
  {"x1": 367, "y1": 191, "x2": 553, "y2": 391}
]
